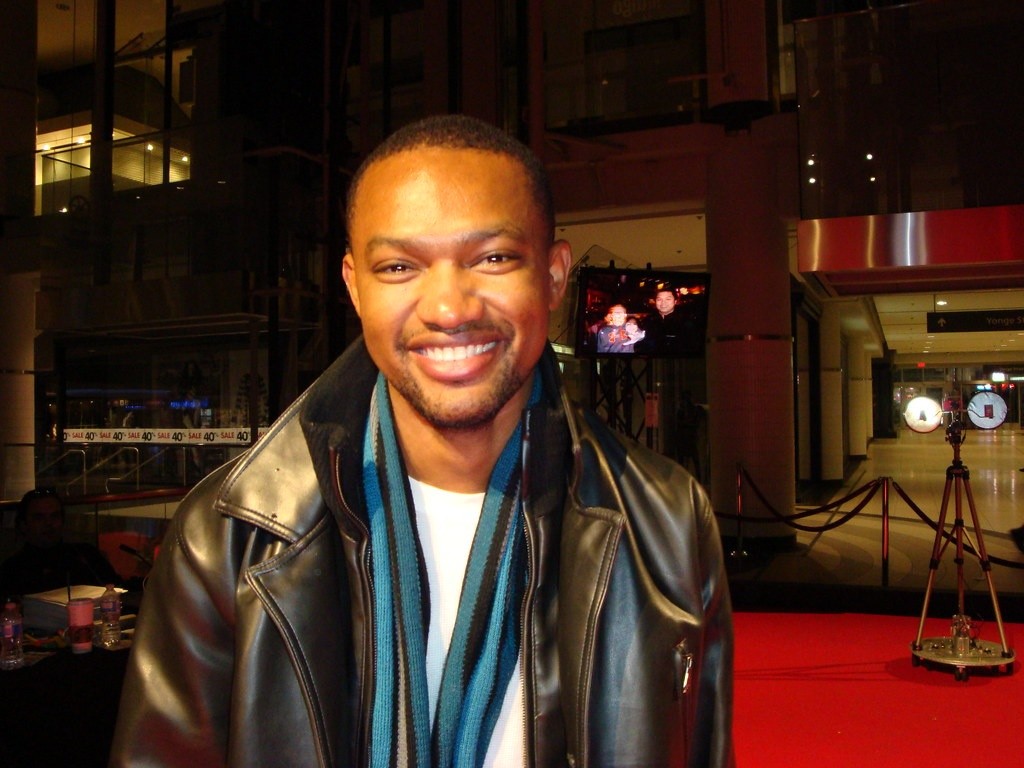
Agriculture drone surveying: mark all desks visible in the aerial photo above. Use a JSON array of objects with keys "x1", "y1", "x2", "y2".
[{"x1": 0, "y1": 619, "x2": 137, "y2": 768}]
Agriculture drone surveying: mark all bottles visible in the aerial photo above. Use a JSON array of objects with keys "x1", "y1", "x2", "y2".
[
  {"x1": 0, "y1": 604, "x2": 23, "y2": 665},
  {"x1": 100, "y1": 584, "x2": 121, "y2": 645}
]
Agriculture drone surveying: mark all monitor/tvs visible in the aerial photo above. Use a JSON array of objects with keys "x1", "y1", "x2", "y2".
[{"x1": 574, "y1": 265, "x2": 709, "y2": 357}]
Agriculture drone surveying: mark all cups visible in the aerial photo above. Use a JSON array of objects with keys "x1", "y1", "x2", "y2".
[{"x1": 67, "y1": 597, "x2": 94, "y2": 654}]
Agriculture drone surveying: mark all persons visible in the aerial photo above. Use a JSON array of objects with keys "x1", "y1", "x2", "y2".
[
  {"x1": 116, "y1": 112, "x2": 735, "y2": 768},
  {"x1": 586, "y1": 285, "x2": 703, "y2": 353},
  {"x1": 0, "y1": 490, "x2": 136, "y2": 616}
]
